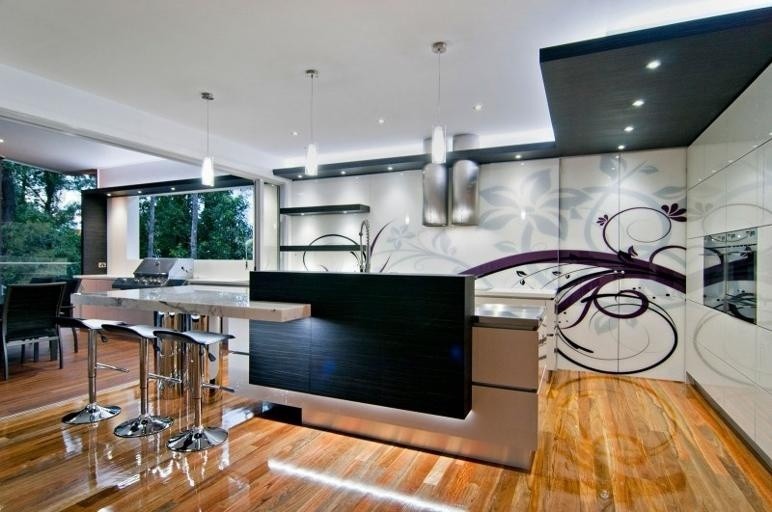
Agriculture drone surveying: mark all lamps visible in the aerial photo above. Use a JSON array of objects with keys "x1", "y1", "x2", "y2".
[{"x1": 200, "y1": 41, "x2": 448, "y2": 187}]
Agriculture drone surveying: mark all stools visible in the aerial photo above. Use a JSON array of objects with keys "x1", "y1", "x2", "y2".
[{"x1": 54, "y1": 314, "x2": 236, "y2": 453}]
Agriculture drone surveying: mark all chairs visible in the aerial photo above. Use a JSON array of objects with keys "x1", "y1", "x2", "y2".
[{"x1": 0, "y1": 276, "x2": 78, "y2": 379}]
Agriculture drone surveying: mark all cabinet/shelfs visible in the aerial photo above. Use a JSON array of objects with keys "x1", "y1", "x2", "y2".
[{"x1": 279, "y1": 205, "x2": 371, "y2": 251}]
